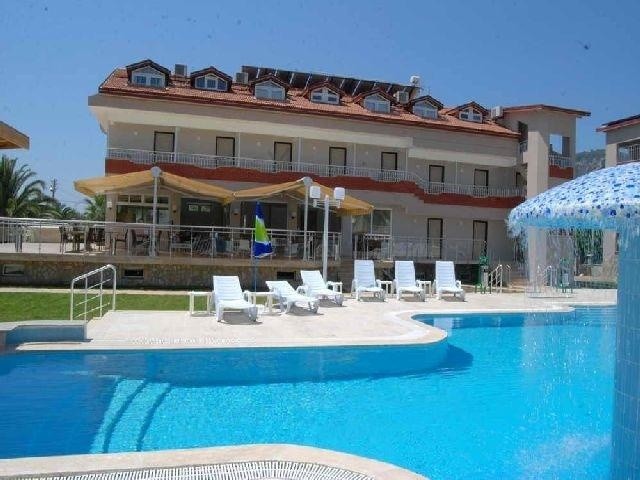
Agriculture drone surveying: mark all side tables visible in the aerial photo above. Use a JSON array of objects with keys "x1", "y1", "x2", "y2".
[
  {"x1": 326, "y1": 281, "x2": 343, "y2": 293},
  {"x1": 380, "y1": 280, "x2": 393, "y2": 297},
  {"x1": 189, "y1": 291, "x2": 212, "y2": 316},
  {"x1": 417, "y1": 280, "x2": 432, "y2": 298},
  {"x1": 250, "y1": 292, "x2": 274, "y2": 314}
]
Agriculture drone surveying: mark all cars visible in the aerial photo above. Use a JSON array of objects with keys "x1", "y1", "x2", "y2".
[{"x1": 67, "y1": 225, "x2": 105, "y2": 243}]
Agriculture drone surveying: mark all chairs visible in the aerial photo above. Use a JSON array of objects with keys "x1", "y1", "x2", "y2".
[
  {"x1": 296, "y1": 270, "x2": 342, "y2": 306},
  {"x1": 212, "y1": 274, "x2": 258, "y2": 321},
  {"x1": 432, "y1": 261, "x2": 464, "y2": 302},
  {"x1": 392, "y1": 261, "x2": 424, "y2": 302},
  {"x1": 265, "y1": 280, "x2": 319, "y2": 312},
  {"x1": 350, "y1": 259, "x2": 384, "y2": 302},
  {"x1": 59, "y1": 222, "x2": 340, "y2": 259}
]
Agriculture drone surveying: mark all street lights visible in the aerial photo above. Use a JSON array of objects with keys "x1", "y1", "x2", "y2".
[
  {"x1": 150, "y1": 166, "x2": 161, "y2": 257},
  {"x1": 301, "y1": 177, "x2": 313, "y2": 260},
  {"x1": 310, "y1": 186, "x2": 345, "y2": 283},
  {"x1": 49, "y1": 178, "x2": 58, "y2": 199}
]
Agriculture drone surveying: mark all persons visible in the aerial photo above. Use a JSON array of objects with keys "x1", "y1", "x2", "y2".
[{"x1": 133, "y1": 218, "x2": 160, "y2": 256}]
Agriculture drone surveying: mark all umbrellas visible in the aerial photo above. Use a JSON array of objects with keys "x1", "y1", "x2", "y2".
[
  {"x1": 505, "y1": 158, "x2": 640, "y2": 239},
  {"x1": 252, "y1": 200, "x2": 272, "y2": 260}
]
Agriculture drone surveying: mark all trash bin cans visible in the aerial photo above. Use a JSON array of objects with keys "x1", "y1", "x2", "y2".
[
  {"x1": 480, "y1": 265, "x2": 489, "y2": 289},
  {"x1": 546, "y1": 266, "x2": 552, "y2": 286},
  {"x1": 561, "y1": 268, "x2": 574, "y2": 292}
]
